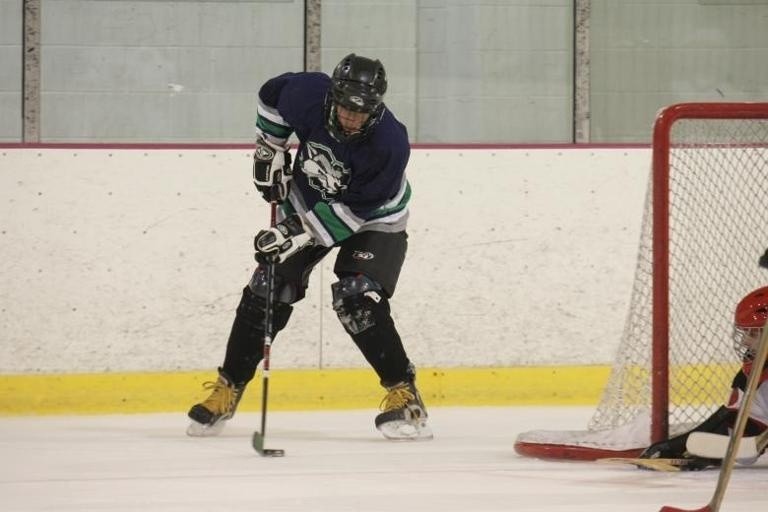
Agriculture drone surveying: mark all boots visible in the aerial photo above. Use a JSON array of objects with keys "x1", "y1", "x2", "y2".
[
  {"x1": 190, "y1": 367, "x2": 246, "y2": 425},
  {"x1": 376, "y1": 374, "x2": 428, "y2": 428}
]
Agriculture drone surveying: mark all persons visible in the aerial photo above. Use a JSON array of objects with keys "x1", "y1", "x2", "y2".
[
  {"x1": 635, "y1": 249, "x2": 768, "y2": 470},
  {"x1": 189, "y1": 52, "x2": 428, "y2": 430}
]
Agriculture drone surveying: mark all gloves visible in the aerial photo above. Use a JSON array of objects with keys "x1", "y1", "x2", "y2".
[
  {"x1": 252, "y1": 131, "x2": 294, "y2": 205},
  {"x1": 254, "y1": 213, "x2": 317, "y2": 265}
]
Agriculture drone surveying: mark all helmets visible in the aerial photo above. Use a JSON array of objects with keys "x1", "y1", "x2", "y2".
[
  {"x1": 322, "y1": 53, "x2": 387, "y2": 145},
  {"x1": 732, "y1": 285, "x2": 768, "y2": 383}
]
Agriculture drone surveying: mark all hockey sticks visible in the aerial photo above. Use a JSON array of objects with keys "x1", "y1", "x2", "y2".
[
  {"x1": 660, "y1": 324, "x2": 768, "y2": 512},
  {"x1": 253, "y1": 201, "x2": 282, "y2": 455}
]
